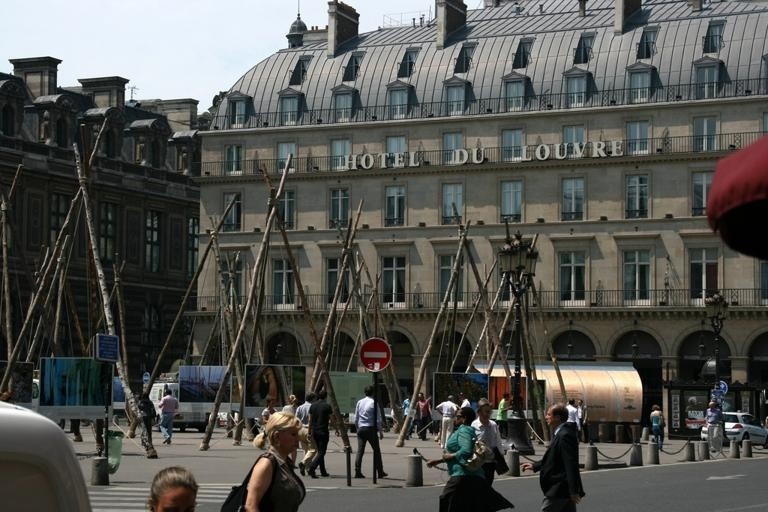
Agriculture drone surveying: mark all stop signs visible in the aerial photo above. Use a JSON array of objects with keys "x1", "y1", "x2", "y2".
[{"x1": 359, "y1": 336, "x2": 393, "y2": 373}]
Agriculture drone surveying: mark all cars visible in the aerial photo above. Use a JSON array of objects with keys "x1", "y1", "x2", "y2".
[
  {"x1": 699, "y1": 408, "x2": 768, "y2": 449},
  {"x1": 28, "y1": 378, "x2": 66, "y2": 429}
]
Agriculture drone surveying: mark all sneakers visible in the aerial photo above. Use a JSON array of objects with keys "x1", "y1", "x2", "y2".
[
  {"x1": 163, "y1": 437, "x2": 172, "y2": 445},
  {"x1": 299, "y1": 462, "x2": 391, "y2": 479}
]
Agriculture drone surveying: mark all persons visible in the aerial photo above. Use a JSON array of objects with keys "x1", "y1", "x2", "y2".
[
  {"x1": 354, "y1": 385, "x2": 590, "y2": 511},
  {"x1": 58, "y1": 386, "x2": 342, "y2": 511},
  {"x1": 649, "y1": 402, "x2": 666, "y2": 451},
  {"x1": 704, "y1": 401, "x2": 724, "y2": 453},
  {"x1": 686, "y1": 396, "x2": 699, "y2": 414}
]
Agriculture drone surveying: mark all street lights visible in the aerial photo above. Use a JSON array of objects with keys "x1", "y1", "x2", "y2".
[
  {"x1": 498, "y1": 229, "x2": 541, "y2": 457},
  {"x1": 703, "y1": 290, "x2": 731, "y2": 446}
]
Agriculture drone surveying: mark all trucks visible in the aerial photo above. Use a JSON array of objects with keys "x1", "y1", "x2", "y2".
[{"x1": 140, "y1": 379, "x2": 209, "y2": 435}]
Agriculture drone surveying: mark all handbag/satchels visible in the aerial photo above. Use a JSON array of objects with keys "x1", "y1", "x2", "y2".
[
  {"x1": 220, "y1": 453, "x2": 273, "y2": 512},
  {"x1": 465, "y1": 441, "x2": 493, "y2": 467},
  {"x1": 491, "y1": 447, "x2": 509, "y2": 476}
]
